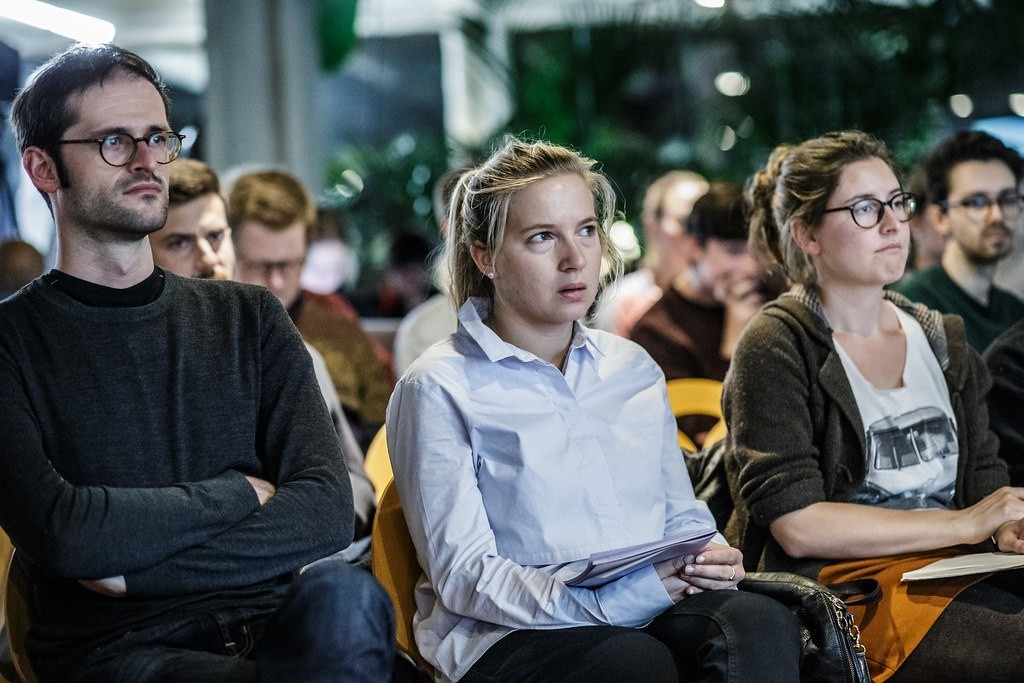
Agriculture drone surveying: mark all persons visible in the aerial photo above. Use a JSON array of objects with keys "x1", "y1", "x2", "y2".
[
  {"x1": 720, "y1": 131, "x2": 1024, "y2": 683},
  {"x1": 385, "y1": 140, "x2": 800, "y2": 682},
  {"x1": 147, "y1": 157, "x2": 375, "y2": 535},
  {"x1": 884, "y1": 130, "x2": 1024, "y2": 485},
  {"x1": 306, "y1": 169, "x2": 467, "y2": 368},
  {"x1": 0, "y1": 40, "x2": 398, "y2": 683},
  {"x1": 226, "y1": 171, "x2": 393, "y2": 454},
  {"x1": 0, "y1": 240, "x2": 43, "y2": 301},
  {"x1": 595, "y1": 168, "x2": 795, "y2": 530}
]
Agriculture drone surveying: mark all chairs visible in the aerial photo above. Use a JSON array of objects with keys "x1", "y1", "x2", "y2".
[
  {"x1": 367, "y1": 422, "x2": 436, "y2": 680},
  {"x1": 667, "y1": 379, "x2": 728, "y2": 452}
]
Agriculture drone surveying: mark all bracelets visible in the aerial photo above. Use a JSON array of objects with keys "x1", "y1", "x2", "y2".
[{"x1": 990, "y1": 535, "x2": 999, "y2": 552}]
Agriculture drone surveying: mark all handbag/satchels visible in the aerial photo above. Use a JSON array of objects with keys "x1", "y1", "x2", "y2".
[{"x1": 736, "y1": 571, "x2": 883, "y2": 683}]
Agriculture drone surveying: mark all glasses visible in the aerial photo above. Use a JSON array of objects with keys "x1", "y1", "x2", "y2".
[
  {"x1": 822, "y1": 192, "x2": 918, "y2": 229},
  {"x1": 944, "y1": 192, "x2": 1024, "y2": 221},
  {"x1": 38, "y1": 131, "x2": 186, "y2": 167}
]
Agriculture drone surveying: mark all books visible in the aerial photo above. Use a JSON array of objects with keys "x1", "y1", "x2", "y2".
[
  {"x1": 551, "y1": 528, "x2": 717, "y2": 586},
  {"x1": 901, "y1": 551, "x2": 1024, "y2": 581}
]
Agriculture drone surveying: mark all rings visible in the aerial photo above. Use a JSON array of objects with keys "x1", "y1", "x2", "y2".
[{"x1": 728, "y1": 565, "x2": 736, "y2": 580}]
